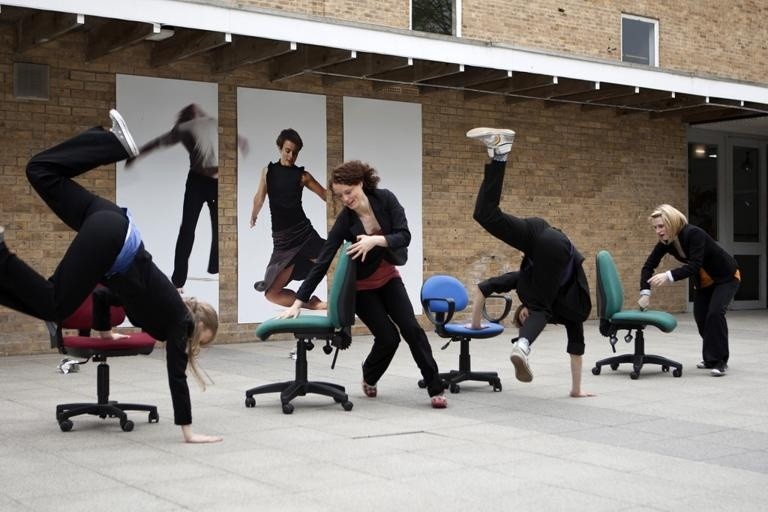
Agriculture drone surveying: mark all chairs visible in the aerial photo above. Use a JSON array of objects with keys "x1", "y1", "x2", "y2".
[
  {"x1": 421, "y1": 275, "x2": 510, "y2": 393},
  {"x1": 245, "y1": 238, "x2": 358, "y2": 418},
  {"x1": 57, "y1": 271, "x2": 159, "y2": 431},
  {"x1": 594, "y1": 251, "x2": 683, "y2": 378}
]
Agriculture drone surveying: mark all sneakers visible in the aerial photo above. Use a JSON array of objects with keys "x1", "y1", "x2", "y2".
[
  {"x1": 510, "y1": 341, "x2": 533, "y2": 382},
  {"x1": 710, "y1": 368, "x2": 725, "y2": 377},
  {"x1": 466, "y1": 127, "x2": 517, "y2": 159},
  {"x1": 696, "y1": 361, "x2": 707, "y2": 369},
  {"x1": 108, "y1": 108, "x2": 139, "y2": 159},
  {"x1": 363, "y1": 380, "x2": 377, "y2": 398},
  {"x1": 431, "y1": 393, "x2": 447, "y2": 408}
]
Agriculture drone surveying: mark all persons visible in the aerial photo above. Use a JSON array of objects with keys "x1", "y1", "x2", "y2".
[
  {"x1": 123, "y1": 102, "x2": 252, "y2": 297},
  {"x1": 0, "y1": 108, "x2": 227, "y2": 444},
  {"x1": 246, "y1": 127, "x2": 328, "y2": 310},
  {"x1": 637, "y1": 205, "x2": 742, "y2": 376},
  {"x1": 272, "y1": 161, "x2": 449, "y2": 408},
  {"x1": 464, "y1": 126, "x2": 599, "y2": 399}
]
